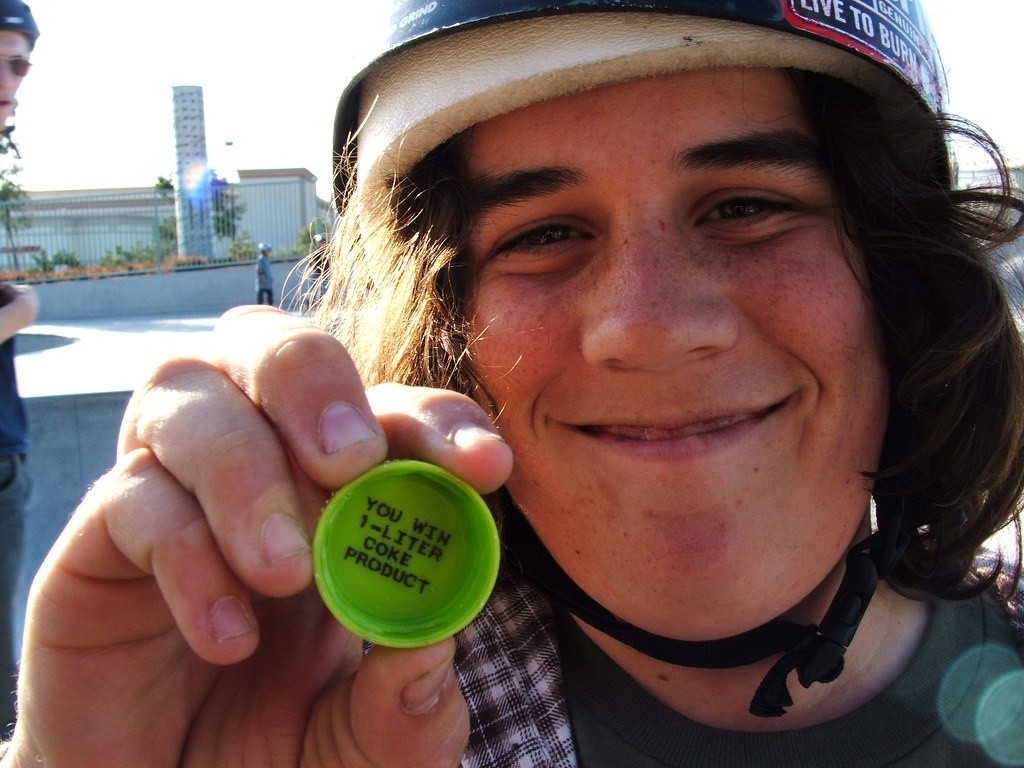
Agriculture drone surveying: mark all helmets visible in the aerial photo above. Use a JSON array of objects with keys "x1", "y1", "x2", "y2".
[
  {"x1": 315, "y1": 235, "x2": 330, "y2": 248},
  {"x1": 333, "y1": 0, "x2": 953, "y2": 218},
  {"x1": 259, "y1": 242, "x2": 272, "y2": 253}
]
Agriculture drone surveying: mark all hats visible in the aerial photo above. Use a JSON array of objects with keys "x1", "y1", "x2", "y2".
[{"x1": 0, "y1": 0, "x2": 40, "y2": 52}]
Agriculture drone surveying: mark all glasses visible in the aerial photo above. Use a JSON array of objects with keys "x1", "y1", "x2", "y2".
[{"x1": 0, "y1": 58, "x2": 34, "y2": 76}]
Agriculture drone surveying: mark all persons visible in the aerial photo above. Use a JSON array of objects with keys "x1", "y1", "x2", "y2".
[
  {"x1": 0, "y1": 0, "x2": 1024, "y2": 768},
  {"x1": 256, "y1": 242, "x2": 273, "y2": 305},
  {"x1": 0, "y1": 0, "x2": 39, "y2": 742},
  {"x1": 302, "y1": 224, "x2": 331, "y2": 314}
]
